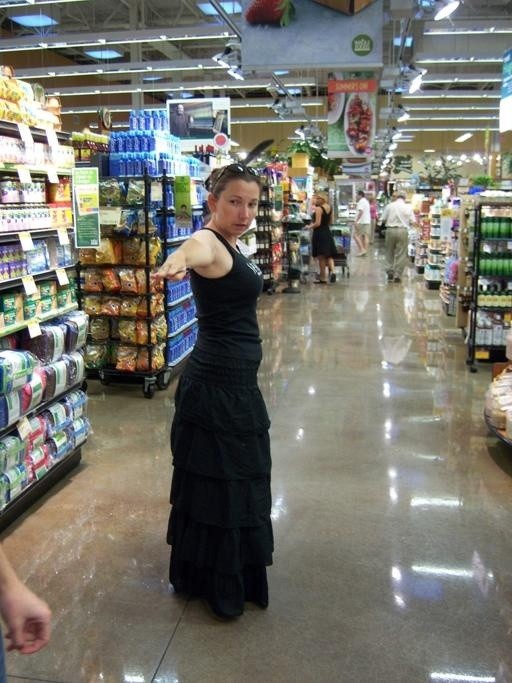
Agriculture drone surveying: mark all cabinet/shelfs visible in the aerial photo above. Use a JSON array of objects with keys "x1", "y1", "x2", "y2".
[
  {"x1": 466, "y1": 198, "x2": 512, "y2": 374},
  {"x1": 0, "y1": 118, "x2": 95, "y2": 534},
  {"x1": 250, "y1": 169, "x2": 315, "y2": 296},
  {"x1": 408, "y1": 185, "x2": 512, "y2": 341},
  {"x1": 75, "y1": 148, "x2": 173, "y2": 400},
  {"x1": 100, "y1": 174, "x2": 205, "y2": 369}
]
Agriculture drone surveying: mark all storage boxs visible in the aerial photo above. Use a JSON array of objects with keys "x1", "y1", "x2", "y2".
[{"x1": 290, "y1": 152, "x2": 311, "y2": 169}]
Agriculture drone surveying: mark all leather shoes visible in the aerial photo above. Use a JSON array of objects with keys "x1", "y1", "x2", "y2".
[
  {"x1": 394, "y1": 278, "x2": 401, "y2": 282},
  {"x1": 388, "y1": 274, "x2": 393, "y2": 279}
]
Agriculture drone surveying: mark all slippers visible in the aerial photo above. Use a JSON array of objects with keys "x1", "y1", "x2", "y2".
[
  {"x1": 330, "y1": 273, "x2": 336, "y2": 282},
  {"x1": 313, "y1": 279, "x2": 327, "y2": 283}
]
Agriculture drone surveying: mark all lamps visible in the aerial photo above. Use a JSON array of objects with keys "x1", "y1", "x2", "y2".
[
  {"x1": 165, "y1": 91, "x2": 195, "y2": 99},
  {"x1": 212, "y1": 49, "x2": 240, "y2": 68},
  {"x1": 396, "y1": 58, "x2": 423, "y2": 95},
  {"x1": 194, "y1": 0, "x2": 246, "y2": 16},
  {"x1": 81, "y1": 43, "x2": 125, "y2": 60},
  {"x1": 366, "y1": 126, "x2": 402, "y2": 179},
  {"x1": 142, "y1": 75, "x2": 164, "y2": 81},
  {"x1": 378, "y1": 104, "x2": 411, "y2": 123},
  {"x1": 226, "y1": 65, "x2": 253, "y2": 81},
  {"x1": 274, "y1": 86, "x2": 303, "y2": 95},
  {"x1": 272, "y1": 69, "x2": 290, "y2": 76},
  {"x1": 269, "y1": 95, "x2": 306, "y2": 120},
  {"x1": 6, "y1": 3, "x2": 63, "y2": 29},
  {"x1": 294, "y1": 122, "x2": 328, "y2": 160}
]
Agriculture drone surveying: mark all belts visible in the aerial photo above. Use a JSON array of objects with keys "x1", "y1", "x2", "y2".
[{"x1": 387, "y1": 226, "x2": 407, "y2": 229}]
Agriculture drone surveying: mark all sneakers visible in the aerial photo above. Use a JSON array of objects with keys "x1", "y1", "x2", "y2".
[{"x1": 357, "y1": 249, "x2": 367, "y2": 257}]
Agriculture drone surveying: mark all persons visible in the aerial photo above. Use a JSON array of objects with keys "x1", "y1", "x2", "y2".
[
  {"x1": 303, "y1": 194, "x2": 338, "y2": 284},
  {"x1": 368, "y1": 196, "x2": 379, "y2": 245},
  {"x1": 0, "y1": 546, "x2": 52, "y2": 683},
  {"x1": 150, "y1": 164, "x2": 274, "y2": 621},
  {"x1": 170, "y1": 103, "x2": 193, "y2": 137},
  {"x1": 353, "y1": 190, "x2": 371, "y2": 257},
  {"x1": 375, "y1": 191, "x2": 417, "y2": 282}
]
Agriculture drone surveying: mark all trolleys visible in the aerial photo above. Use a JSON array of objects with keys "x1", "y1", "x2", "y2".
[{"x1": 325, "y1": 224, "x2": 352, "y2": 279}]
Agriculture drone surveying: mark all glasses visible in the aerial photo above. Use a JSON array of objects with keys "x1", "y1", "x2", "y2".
[
  {"x1": 211, "y1": 163, "x2": 259, "y2": 194},
  {"x1": 316, "y1": 198, "x2": 322, "y2": 200}
]
